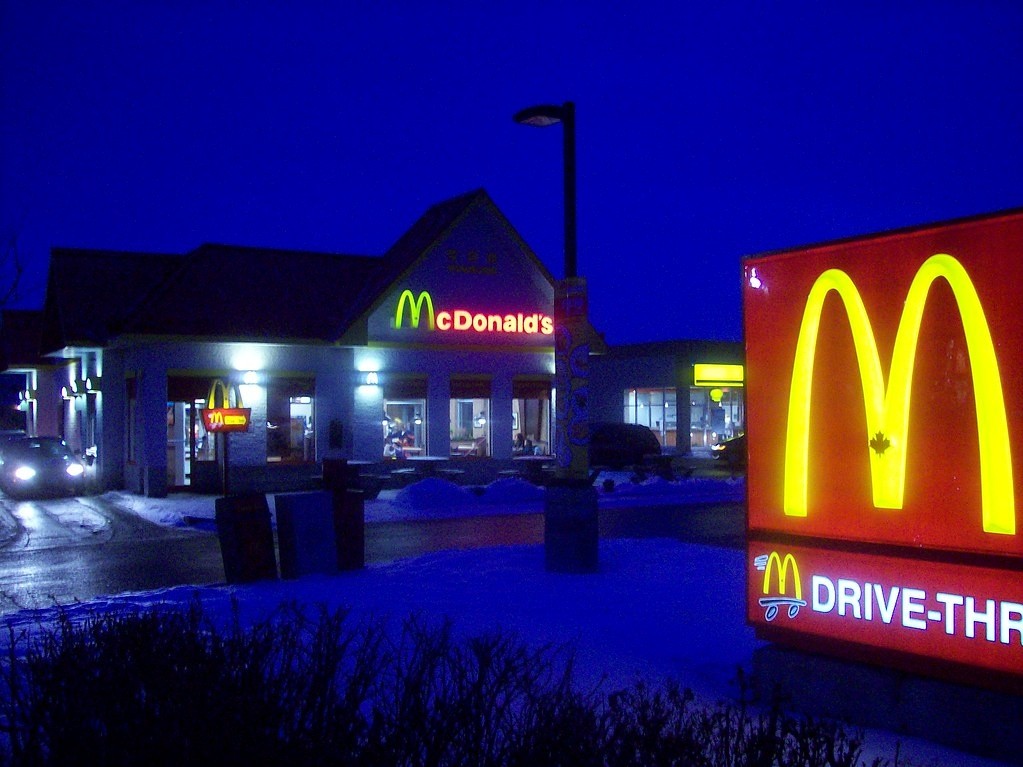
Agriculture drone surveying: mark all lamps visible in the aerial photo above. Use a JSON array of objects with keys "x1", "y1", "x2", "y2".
[
  {"x1": 381, "y1": 413, "x2": 392, "y2": 426},
  {"x1": 414, "y1": 412, "x2": 423, "y2": 425},
  {"x1": 60, "y1": 375, "x2": 102, "y2": 399},
  {"x1": 478, "y1": 411, "x2": 487, "y2": 424},
  {"x1": 18, "y1": 389, "x2": 38, "y2": 401}
]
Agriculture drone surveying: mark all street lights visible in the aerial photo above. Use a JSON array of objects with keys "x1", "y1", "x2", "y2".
[{"x1": 514, "y1": 101, "x2": 579, "y2": 277}]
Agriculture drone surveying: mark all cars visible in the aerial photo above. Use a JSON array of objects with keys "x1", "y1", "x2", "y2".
[
  {"x1": 0, "y1": 435, "x2": 85, "y2": 497},
  {"x1": 712, "y1": 434, "x2": 747, "y2": 471},
  {"x1": 590, "y1": 422, "x2": 665, "y2": 471},
  {"x1": 0, "y1": 429, "x2": 27, "y2": 443}
]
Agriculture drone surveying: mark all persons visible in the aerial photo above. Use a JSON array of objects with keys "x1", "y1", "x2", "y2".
[
  {"x1": 515, "y1": 433, "x2": 524, "y2": 456},
  {"x1": 185, "y1": 424, "x2": 209, "y2": 460},
  {"x1": 390, "y1": 424, "x2": 414, "y2": 459}
]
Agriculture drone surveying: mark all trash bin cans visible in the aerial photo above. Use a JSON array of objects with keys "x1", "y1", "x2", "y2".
[
  {"x1": 215, "y1": 491, "x2": 278, "y2": 585},
  {"x1": 334, "y1": 487, "x2": 365, "y2": 571},
  {"x1": 274, "y1": 490, "x2": 339, "y2": 579}
]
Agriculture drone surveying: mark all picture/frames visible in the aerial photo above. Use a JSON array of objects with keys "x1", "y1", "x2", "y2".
[
  {"x1": 474, "y1": 416, "x2": 483, "y2": 429},
  {"x1": 512, "y1": 412, "x2": 519, "y2": 430}
]
